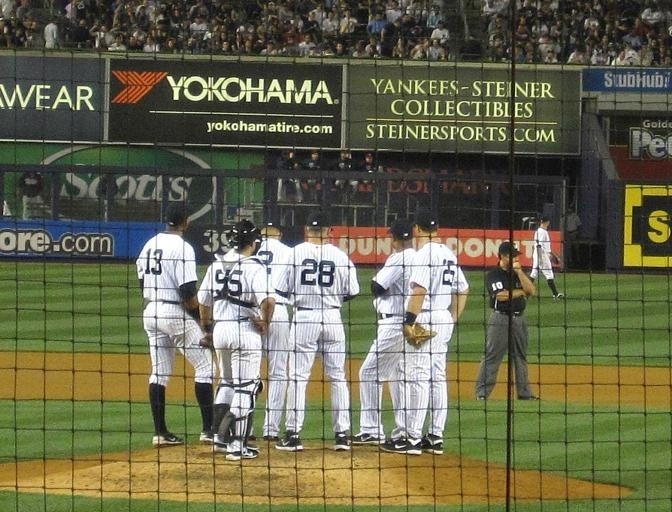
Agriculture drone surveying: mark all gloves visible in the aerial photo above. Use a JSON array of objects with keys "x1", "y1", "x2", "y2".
[{"x1": 554, "y1": 256, "x2": 560, "y2": 264}]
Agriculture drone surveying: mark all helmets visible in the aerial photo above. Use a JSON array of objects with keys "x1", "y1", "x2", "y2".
[
  {"x1": 280, "y1": 209, "x2": 305, "y2": 228},
  {"x1": 226, "y1": 220, "x2": 258, "y2": 250}
]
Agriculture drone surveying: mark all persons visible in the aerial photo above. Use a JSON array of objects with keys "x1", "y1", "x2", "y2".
[
  {"x1": 0, "y1": 0, "x2": 671, "y2": 65},
  {"x1": 526, "y1": 216, "x2": 564, "y2": 299},
  {"x1": 475, "y1": 243, "x2": 541, "y2": 401},
  {"x1": 136, "y1": 201, "x2": 216, "y2": 447},
  {"x1": 194, "y1": 211, "x2": 359, "y2": 462},
  {"x1": 274, "y1": 150, "x2": 378, "y2": 226},
  {"x1": 347, "y1": 207, "x2": 470, "y2": 456},
  {"x1": 564, "y1": 205, "x2": 582, "y2": 266},
  {"x1": 19, "y1": 168, "x2": 62, "y2": 219}
]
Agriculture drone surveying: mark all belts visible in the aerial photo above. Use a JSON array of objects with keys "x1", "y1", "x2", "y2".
[{"x1": 494, "y1": 310, "x2": 522, "y2": 316}]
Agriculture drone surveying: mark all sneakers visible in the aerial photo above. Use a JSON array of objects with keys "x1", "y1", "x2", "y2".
[
  {"x1": 552, "y1": 293, "x2": 565, "y2": 299},
  {"x1": 275, "y1": 430, "x2": 303, "y2": 451},
  {"x1": 380, "y1": 437, "x2": 422, "y2": 454},
  {"x1": 264, "y1": 436, "x2": 279, "y2": 441},
  {"x1": 422, "y1": 434, "x2": 444, "y2": 455},
  {"x1": 152, "y1": 433, "x2": 185, "y2": 446},
  {"x1": 353, "y1": 433, "x2": 386, "y2": 445},
  {"x1": 518, "y1": 396, "x2": 539, "y2": 400},
  {"x1": 335, "y1": 431, "x2": 350, "y2": 451},
  {"x1": 200, "y1": 430, "x2": 259, "y2": 460}
]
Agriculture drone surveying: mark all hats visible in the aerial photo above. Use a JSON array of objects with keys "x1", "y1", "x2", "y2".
[
  {"x1": 307, "y1": 210, "x2": 330, "y2": 227},
  {"x1": 499, "y1": 242, "x2": 522, "y2": 257},
  {"x1": 540, "y1": 214, "x2": 552, "y2": 220},
  {"x1": 408, "y1": 207, "x2": 438, "y2": 226},
  {"x1": 165, "y1": 202, "x2": 186, "y2": 225},
  {"x1": 387, "y1": 219, "x2": 413, "y2": 236}
]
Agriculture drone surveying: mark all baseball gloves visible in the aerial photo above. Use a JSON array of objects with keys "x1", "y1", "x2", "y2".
[
  {"x1": 550, "y1": 252, "x2": 559, "y2": 264},
  {"x1": 411, "y1": 324, "x2": 436, "y2": 348}
]
